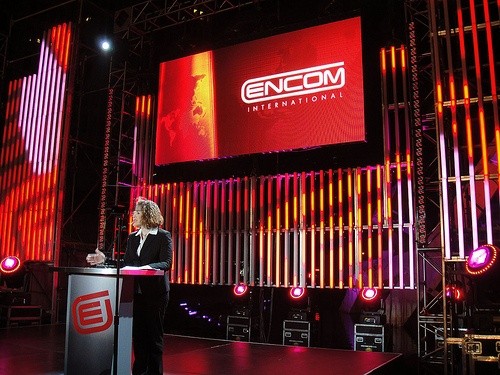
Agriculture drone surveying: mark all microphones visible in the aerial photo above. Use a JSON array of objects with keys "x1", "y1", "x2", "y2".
[{"x1": 104, "y1": 225, "x2": 126, "y2": 265}]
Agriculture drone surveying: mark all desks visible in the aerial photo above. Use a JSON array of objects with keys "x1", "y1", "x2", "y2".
[{"x1": 0, "y1": 306, "x2": 42, "y2": 333}]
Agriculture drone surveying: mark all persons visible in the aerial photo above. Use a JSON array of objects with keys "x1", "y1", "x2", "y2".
[{"x1": 86, "y1": 198, "x2": 173, "y2": 375}]
